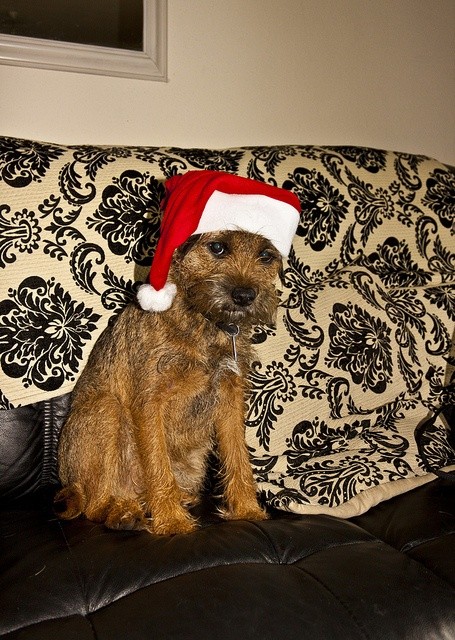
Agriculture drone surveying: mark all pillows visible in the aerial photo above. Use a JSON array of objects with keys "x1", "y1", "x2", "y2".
[
  {"x1": 244, "y1": 255, "x2": 430, "y2": 455},
  {"x1": 42, "y1": 314, "x2": 117, "y2": 488},
  {"x1": 386, "y1": 283, "x2": 455, "y2": 395}
]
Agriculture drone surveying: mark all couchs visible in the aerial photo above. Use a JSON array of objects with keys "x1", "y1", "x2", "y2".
[{"x1": 0, "y1": 137, "x2": 455, "y2": 640}]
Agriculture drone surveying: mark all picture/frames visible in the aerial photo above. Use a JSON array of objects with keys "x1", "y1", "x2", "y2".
[{"x1": 0, "y1": 0, "x2": 168, "y2": 83}]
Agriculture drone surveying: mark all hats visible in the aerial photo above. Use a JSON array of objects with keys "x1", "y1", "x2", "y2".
[{"x1": 140, "y1": 171, "x2": 303, "y2": 308}]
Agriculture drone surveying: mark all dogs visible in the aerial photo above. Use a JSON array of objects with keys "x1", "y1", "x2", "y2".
[{"x1": 51, "y1": 170, "x2": 303, "y2": 539}]
